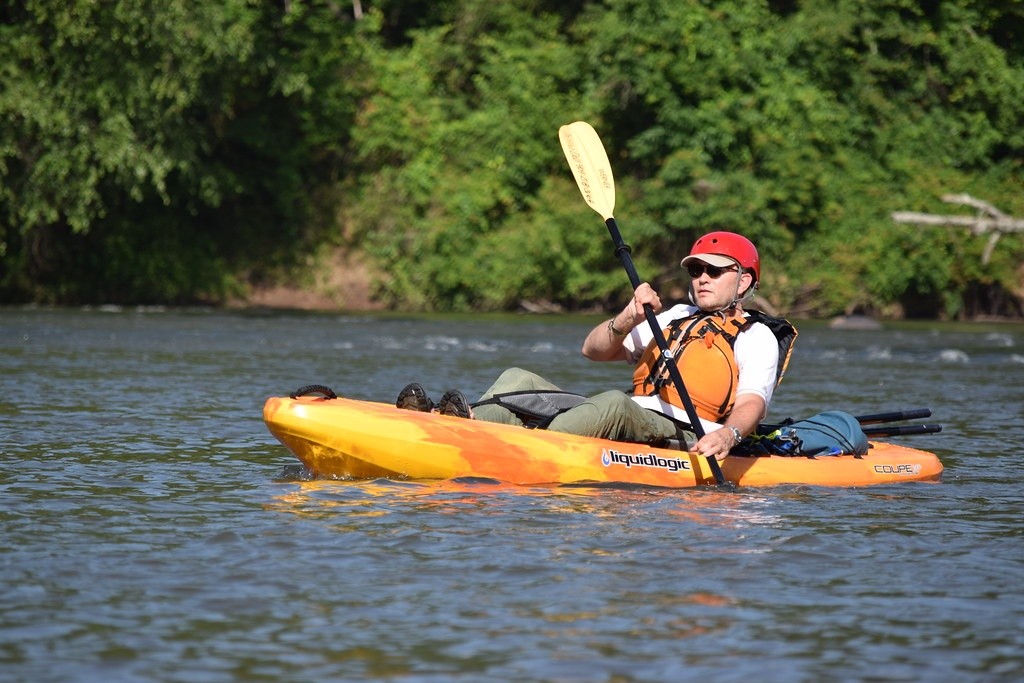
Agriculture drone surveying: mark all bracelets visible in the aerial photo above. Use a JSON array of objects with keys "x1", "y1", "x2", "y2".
[{"x1": 608, "y1": 316, "x2": 629, "y2": 335}]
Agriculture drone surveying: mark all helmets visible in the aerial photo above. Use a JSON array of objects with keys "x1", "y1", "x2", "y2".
[{"x1": 680, "y1": 231, "x2": 760, "y2": 289}]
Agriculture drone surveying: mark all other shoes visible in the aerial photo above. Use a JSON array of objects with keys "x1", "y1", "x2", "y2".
[
  {"x1": 396, "y1": 382, "x2": 431, "y2": 413},
  {"x1": 439, "y1": 389, "x2": 473, "y2": 419}
]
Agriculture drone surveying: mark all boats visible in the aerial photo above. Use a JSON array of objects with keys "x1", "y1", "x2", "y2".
[{"x1": 265, "y1": 386, "x2": 945, "y2": 488}]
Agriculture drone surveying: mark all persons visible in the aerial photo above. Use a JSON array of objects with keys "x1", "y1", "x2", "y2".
[{"x1": 397, "y1": 232, "x2": 779, "y2": 460}]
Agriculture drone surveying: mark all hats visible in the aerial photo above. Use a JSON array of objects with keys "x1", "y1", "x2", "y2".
[{"x1": 681, "y1": 254, "x2": 736, "y2": 268}]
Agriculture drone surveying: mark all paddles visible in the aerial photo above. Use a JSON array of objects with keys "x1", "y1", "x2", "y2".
[{"x1": 556, "y1": 121, "x2": 726, "y2": 489}]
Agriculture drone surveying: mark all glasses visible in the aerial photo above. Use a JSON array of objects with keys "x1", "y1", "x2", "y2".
[{"x1": 688, "y1": 262, "x2": 738, "y2": 278}]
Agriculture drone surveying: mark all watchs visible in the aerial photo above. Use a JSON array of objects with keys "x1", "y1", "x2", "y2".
[{"x1": 725, "y1": 425, "x2": 741, "y2": 442}]
